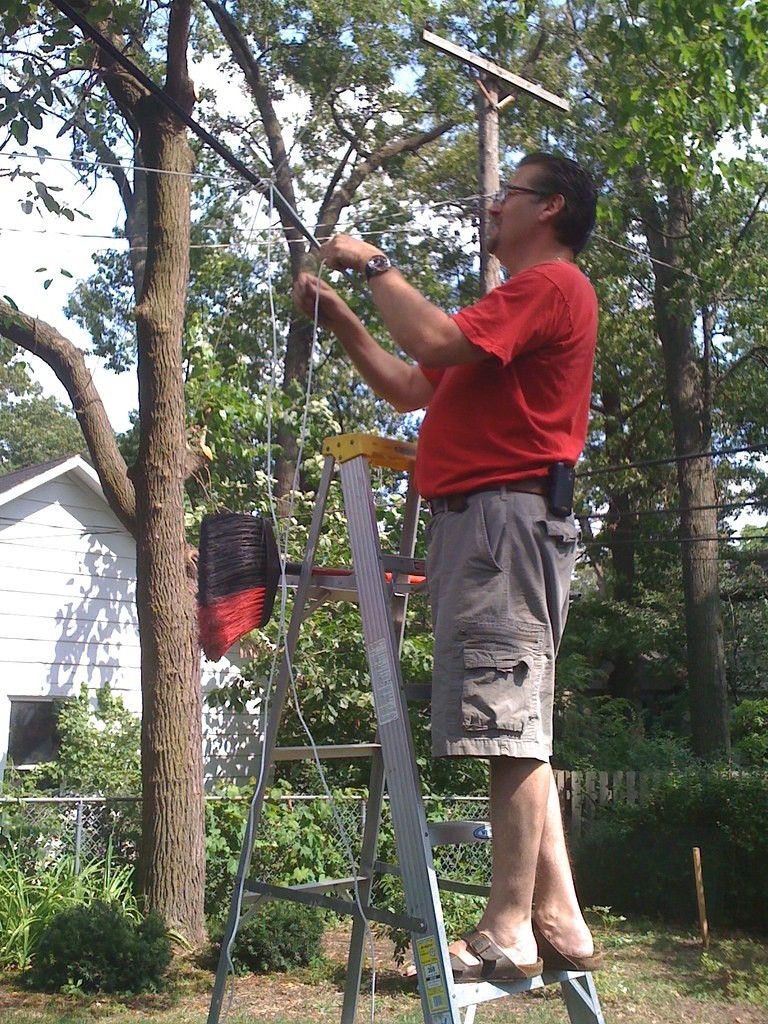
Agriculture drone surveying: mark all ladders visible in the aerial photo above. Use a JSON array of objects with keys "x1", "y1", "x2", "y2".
[{"x1": 208, "y1": 433, "x2": 603, "y2": 1024}]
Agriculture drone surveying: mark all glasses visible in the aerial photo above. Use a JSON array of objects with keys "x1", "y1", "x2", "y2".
[{"x1": 492, "y1": 184, "x2": 541, "y2": 202}]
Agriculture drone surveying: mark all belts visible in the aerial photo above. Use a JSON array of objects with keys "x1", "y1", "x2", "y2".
[{"x1": 426, "y1": 477, "x2": 549, "y2": 513}]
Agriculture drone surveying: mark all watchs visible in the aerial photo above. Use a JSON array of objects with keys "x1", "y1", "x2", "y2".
[{"x1": 366, "y1": 254, "x2": 398, "y2": 281}]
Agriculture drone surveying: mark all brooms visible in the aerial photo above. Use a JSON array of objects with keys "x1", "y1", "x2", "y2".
[{"x1": 194, "y1": 512, "x2": 427, "y2": 664}]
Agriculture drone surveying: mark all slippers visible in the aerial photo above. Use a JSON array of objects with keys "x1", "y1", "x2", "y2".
[
  {"x1": 531, "y1": 917, "x2": 604, "y2": 971},
  {"x1": 401, "y1": 923, "x2": 544, "y2": 984}
]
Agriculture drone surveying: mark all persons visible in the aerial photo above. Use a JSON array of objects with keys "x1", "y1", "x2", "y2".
[{"x1": 292, "y1": 151, "x2": 602, "y2": 983}]
